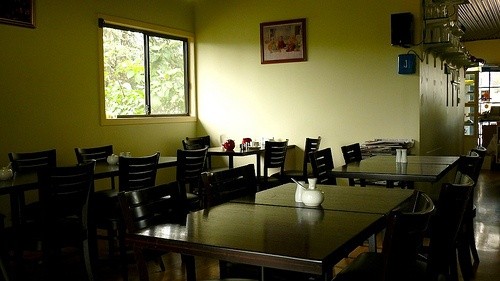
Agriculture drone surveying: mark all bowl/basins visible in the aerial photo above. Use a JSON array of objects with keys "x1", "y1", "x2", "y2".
[{"x1": 107, "y1": 153, "x2": 119, "y2": 165}]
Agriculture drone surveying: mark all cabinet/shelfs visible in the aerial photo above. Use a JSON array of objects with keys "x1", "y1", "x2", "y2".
[
  {"x1": 423, "y1": 0, "x2": 479, "y2": 78},
  {"x1": 464, "y1": 72, "x2": 479, "y2": 155}
]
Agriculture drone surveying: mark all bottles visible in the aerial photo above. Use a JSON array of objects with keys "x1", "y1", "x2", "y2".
[
  {"x1": 240, "y1": 142, "x2": 247, "y2": 150},
  {"x1": 395, "y1": 149, "x2": 408, "y2": 164},
  {"x1": 302, "y1": 178, "x2": 324, "y2": 207},
  {"x1": 120, "y1": 151, "x2": 131, "y2": 158},
  {"x1": 294, "y1": 180, "x2": 305, "y2": 203},
  {"x1": 0, "y1": 167, "x2": 13, "y2": 181}
]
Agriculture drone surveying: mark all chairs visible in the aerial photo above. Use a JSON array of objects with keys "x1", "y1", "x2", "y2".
[
  {"x1": 332, "y1": 143, "x2": 486, "y2": 281},
  {"x1": 0, "y1": 135, "x2": 336, "y2": 281}
]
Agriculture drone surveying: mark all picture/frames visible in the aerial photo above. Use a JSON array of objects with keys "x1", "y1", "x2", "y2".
[
  {"x1": 260, "y1": 18, "x2": 307, "y2": 64},
  {"x1": 0, "y1": 0, "x2": 36, "y2": 29}
]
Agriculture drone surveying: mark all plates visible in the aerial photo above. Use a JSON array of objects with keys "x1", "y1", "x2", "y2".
[{"x1": 248, "y1": 146, "x2": 261, "y2": 149}]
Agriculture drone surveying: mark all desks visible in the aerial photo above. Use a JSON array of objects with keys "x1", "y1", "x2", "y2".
[
  {"x1": 229, "y1": 182, "x2": 416, "y2": 234},
  {"x1": 208, "y1": 147, "x2": 265, "y2": 177},
  {"x1": 0, "y1": 157, "x2": 178, "y2": 227},
  {"x1": 365, "y1": 155, "x2": 461, "y2": 170},
  {"x1": 125, "y1": 201, "x2": 384, "y2": 281},
  {"x1": 332, "y1": 159, "x2": 450, "y2": 189}
]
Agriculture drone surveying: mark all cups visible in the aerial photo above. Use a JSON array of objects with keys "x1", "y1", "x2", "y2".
[{"x1": 250, "y1": 142, "x2": 259, "y2": 146}]
